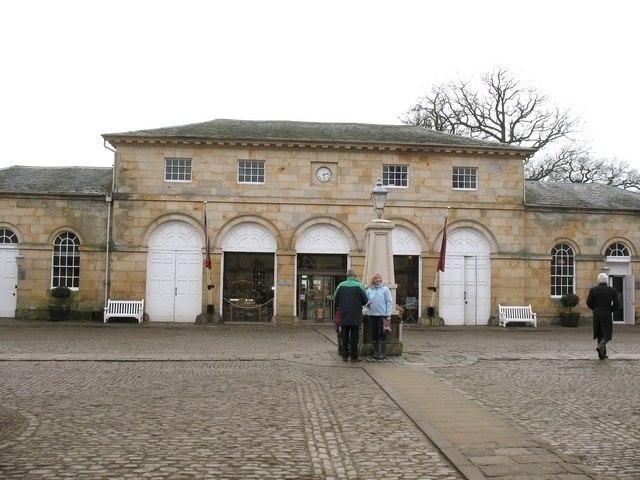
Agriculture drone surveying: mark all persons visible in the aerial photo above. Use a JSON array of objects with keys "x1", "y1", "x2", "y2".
[
  {"x1": 586, "y1": 273, "x2": 619, "y2": 360},
  {"x1": 333, "y1": 268, "x2": 368, "y2": 361},
  {"x1": 365, "y1": 273, "x2": 392, "y2": 359}
]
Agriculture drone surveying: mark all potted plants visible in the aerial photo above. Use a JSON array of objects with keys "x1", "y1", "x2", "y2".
[
  {"x1": 558, "y1": 292, "x2": 582, "y2": 328},
  {"x1": 46, "y1": 284, "x2": 72, "y2": 322}
]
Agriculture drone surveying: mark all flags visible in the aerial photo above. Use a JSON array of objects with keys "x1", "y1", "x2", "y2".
[
  {"x1": 437, "y1": 217, "x2": 446, "y2": 271},
  {"x1": 204, "y1": 211, "x2": 212, "y2": 270}
]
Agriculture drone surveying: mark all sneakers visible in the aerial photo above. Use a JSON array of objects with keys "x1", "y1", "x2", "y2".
[
  {"x1": 352, "y1": 358, "x2": 362, "y2": 362},
  {"x1": 596, "y1": 347, "x2": 603, "y2": 360}
]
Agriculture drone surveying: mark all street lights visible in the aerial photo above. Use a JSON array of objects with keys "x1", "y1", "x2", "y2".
[{"x1": 359, "y1": 177, "x2": 401, "y2": 308}]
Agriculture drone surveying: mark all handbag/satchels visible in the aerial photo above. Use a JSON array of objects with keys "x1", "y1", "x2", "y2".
[
  {"x1": 383, "y1": 318, "x2": 392, "y2": 335},
  {"x1": 335, "y1": 308, "x2": 344, "y2": 327}
]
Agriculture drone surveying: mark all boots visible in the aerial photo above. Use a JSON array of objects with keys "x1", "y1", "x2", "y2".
[{"x1": 371, "y1": 340, "x2": 385, "y2": 359}]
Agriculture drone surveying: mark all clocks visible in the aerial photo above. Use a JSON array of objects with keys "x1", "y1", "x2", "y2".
[{"x1": 315, "y1": 164, "x2": 333, "y2": 184}]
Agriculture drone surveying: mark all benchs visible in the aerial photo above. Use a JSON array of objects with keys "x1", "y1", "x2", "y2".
[
  {"x1": 102, "y1": 298, "x2": 147, "y2": 325},
  {"x1": 497, "y1": 302, "x2": 539, "y2": 329}
]
